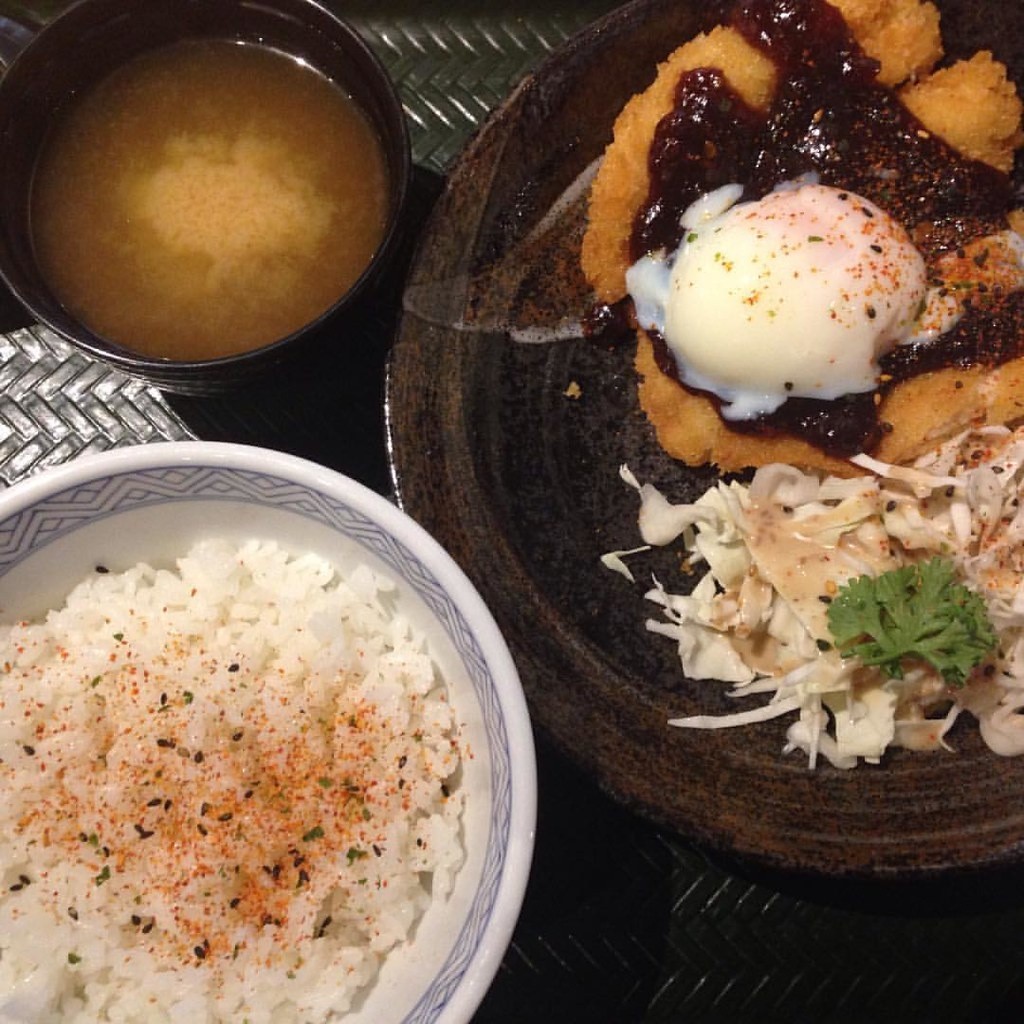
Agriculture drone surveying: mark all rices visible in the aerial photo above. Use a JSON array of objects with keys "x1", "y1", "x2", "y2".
[{"x1": 0, "y1": 539, "x2": 481, "y2": 1022}]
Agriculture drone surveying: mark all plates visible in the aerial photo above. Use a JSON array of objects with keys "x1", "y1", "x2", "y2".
[{"x1": 379, "y1": 3, "x2": 1023, "y2": 884}]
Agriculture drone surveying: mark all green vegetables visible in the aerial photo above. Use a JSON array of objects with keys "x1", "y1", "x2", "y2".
[{"x1": 824, "y1": 558, "x2": 999, "y2": 684}]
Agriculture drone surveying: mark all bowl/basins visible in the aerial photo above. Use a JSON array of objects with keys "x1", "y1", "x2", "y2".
[
  {"x1": 0, "y1": 424, "x2": 534, "y2": 1024},
  {"x1": 1, "y1": 2, "x2": 419, "y2": 400}
]
